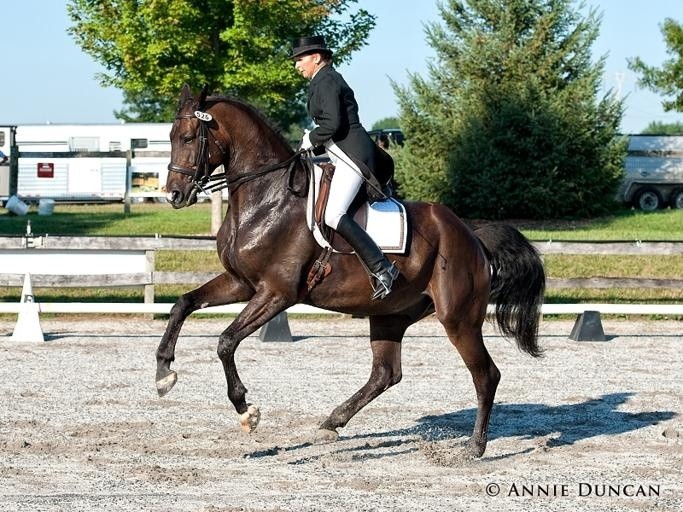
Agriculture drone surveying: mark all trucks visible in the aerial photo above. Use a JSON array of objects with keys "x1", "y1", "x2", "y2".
[
  {"x1": 0, "y1": 122, "x2": 231, "y2": 207},
  {"x1": 609, "y1": 135, "x2": 683, "y2": 210}
]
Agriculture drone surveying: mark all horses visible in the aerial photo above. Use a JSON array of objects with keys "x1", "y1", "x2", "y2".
[{"x1": 156, "y1": 82, "x2": 547, "y2": 469}]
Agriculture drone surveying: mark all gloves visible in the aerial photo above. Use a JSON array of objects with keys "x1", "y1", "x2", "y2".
[{"x1": 298, "y1": 128, "x2": 313, "y2": 151}]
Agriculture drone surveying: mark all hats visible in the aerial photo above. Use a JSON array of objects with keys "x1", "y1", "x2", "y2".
[{"x1": 286, "y1": 36, "x2": 333, "y2": 61}]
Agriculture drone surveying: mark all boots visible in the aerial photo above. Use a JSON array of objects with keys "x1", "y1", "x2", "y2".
[{"x1": 335, "y1": 213, "x2": 400, "y2": 300}]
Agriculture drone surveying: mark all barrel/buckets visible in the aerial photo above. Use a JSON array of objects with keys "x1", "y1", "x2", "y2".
[
  {"x1": 38, "y1": 199, "x2": 55, "y2": 216},
  {"x1": 5, "y1": 195, "x2": 28, "y2": 216}
]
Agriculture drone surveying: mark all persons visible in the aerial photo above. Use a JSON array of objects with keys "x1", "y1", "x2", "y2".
[{"x1": 286, "y1": 34, "x2": 404, "y2": 302}]
